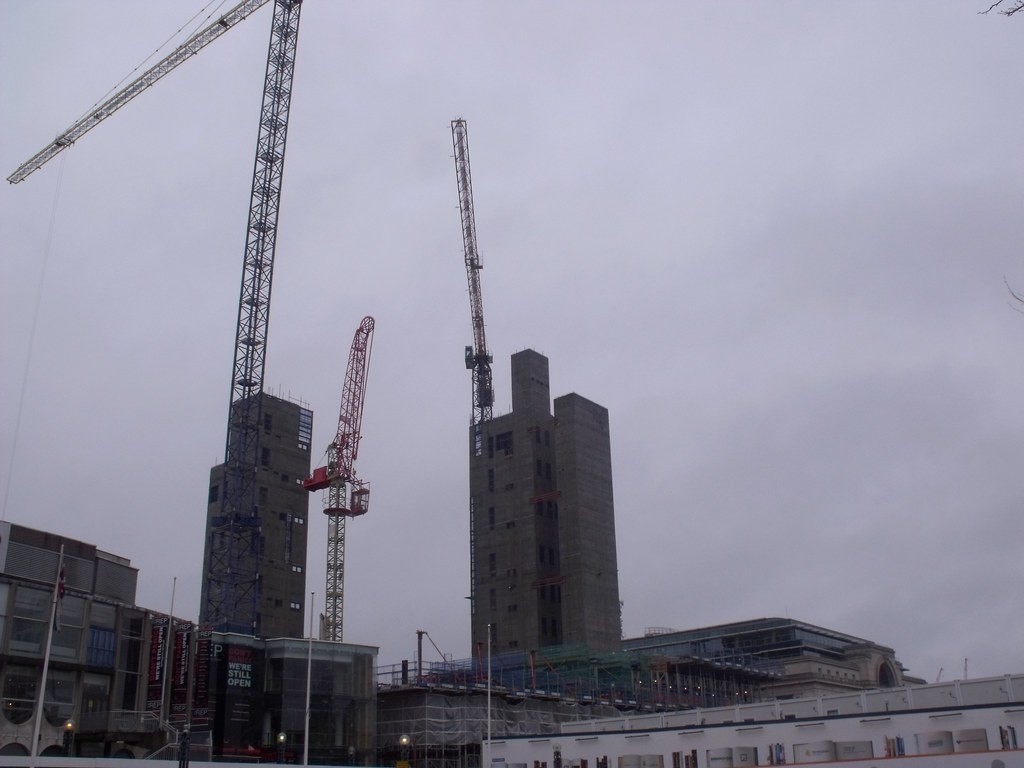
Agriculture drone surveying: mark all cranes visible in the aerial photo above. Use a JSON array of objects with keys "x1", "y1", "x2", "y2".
[
  {"x1": 4, "y1": 0, "x2": 304, "y2": 636},
  {"x1": 303, "y1": 314, "x2": 376, "y2": 641},
  {"x1": 454, "y1": 117, "x2": 496, "y2": 678}
]
